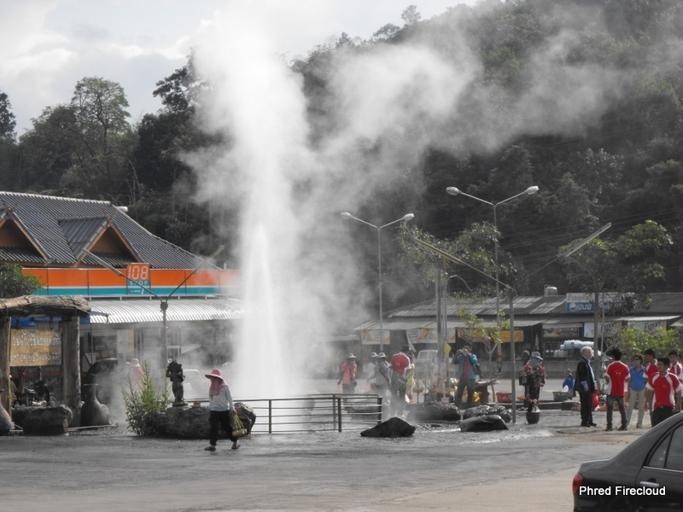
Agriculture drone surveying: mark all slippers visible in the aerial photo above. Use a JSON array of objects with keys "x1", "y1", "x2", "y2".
[
  {"x1": 205, "y1": 445, "x2": 215, "y2": 451},
  {"x1": 232, "y1": 444, "x2": 240, "y2": 449}
]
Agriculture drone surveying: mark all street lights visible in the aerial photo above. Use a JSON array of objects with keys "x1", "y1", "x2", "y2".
[
  {"x1": 447, "y1": 185, "x2": 540, "y2": 372},
  {"x1": 341, "y1": 210, "x2": 415, "y2": 352}
]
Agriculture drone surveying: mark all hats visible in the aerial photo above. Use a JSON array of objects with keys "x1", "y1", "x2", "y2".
[
  {"x1": 348, "y1": 354, "x2": 357, "y2": 359},
  {"x1": 531, "y1": 352, "x2": 543, "y2": 361},
  {"x1": 368, "y1": 352, "x2": 377, "y2": 358},
  {"x1": 378, "y1": 353, "x2": 387, "y2": 359},
  {"x1": 205, "y1": 368, "x2": 223, "y2": 382}
]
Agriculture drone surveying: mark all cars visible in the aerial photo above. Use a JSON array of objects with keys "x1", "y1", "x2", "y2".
[
  {"x1": 573, "y1": 411, "x2": 683, "y2": 512},
  {"x1": 415, "y1": 350, "x2": 438, "y2": 376}
]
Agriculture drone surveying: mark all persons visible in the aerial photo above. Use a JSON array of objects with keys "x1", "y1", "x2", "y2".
[
  {"x1": 200, "y1": 367, "x2": 242, "y2": 451},
  {"x1": 335, "y1": 338, "x2": 683, "y2": 433},
  {"x1": 126, "y1": 357, "x2": 143, "y2": 390}
]
